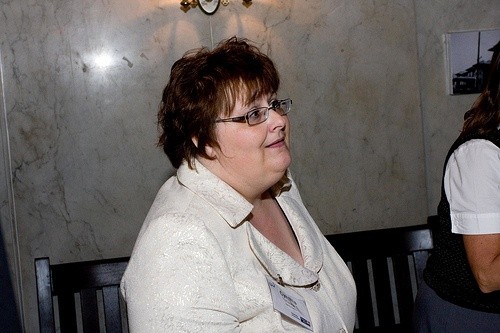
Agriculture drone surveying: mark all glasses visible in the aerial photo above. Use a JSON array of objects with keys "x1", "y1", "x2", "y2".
[{"x1": 213, "y1": 98, "x2": 292, "y2": 125}]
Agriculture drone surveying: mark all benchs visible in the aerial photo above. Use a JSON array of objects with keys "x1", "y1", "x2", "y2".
[{"x1": 34, "y1": 216, "x2": 437, "y2": 333}]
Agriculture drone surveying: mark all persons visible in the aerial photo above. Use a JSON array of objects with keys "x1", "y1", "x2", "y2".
[
  {"x1": 412, "y1": 47, "x2": 500, "y2": 333},
  {"x1": 119, "y1": 36, "x2": 358, "y2": 333}
]
